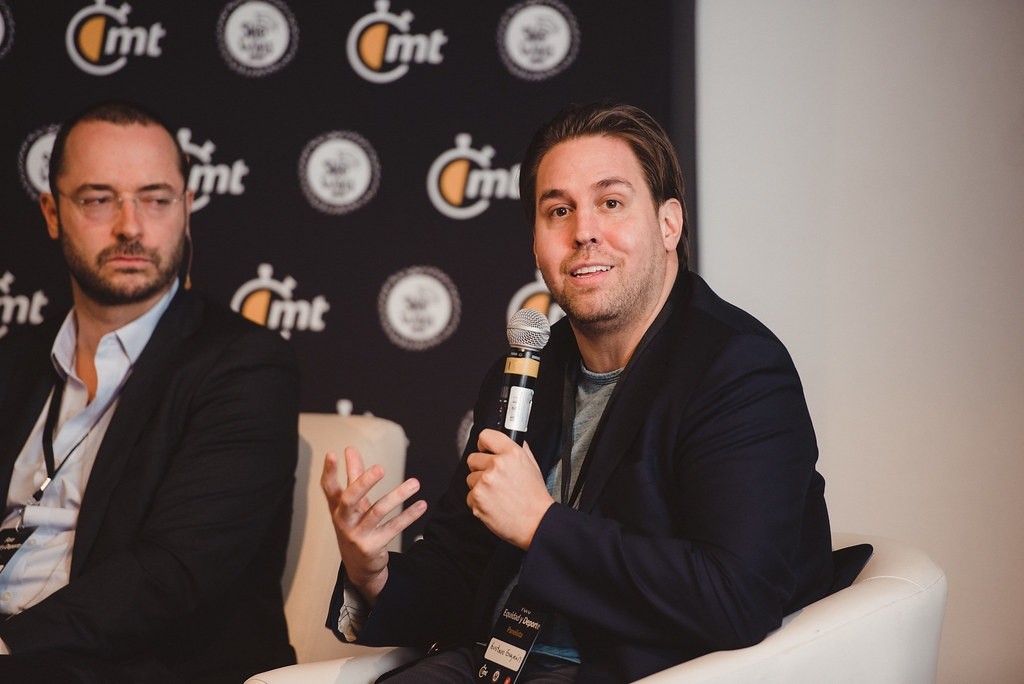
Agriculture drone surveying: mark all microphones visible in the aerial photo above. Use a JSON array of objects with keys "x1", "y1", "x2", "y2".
[{"x1": 487, "y1": 308, "x2": 551, "y2": 456}]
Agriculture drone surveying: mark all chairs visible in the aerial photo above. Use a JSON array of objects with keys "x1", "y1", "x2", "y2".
[
  {"x1": 242, "y1": 534, "x2": 950, "y2": 684},
  {"x1": 281, "y1": 412, "x2": 409, "y2": 664}
]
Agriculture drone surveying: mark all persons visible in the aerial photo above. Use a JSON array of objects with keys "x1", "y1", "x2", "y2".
[
  {"x1": 320, "y1": 100, "x2": 833, "y2": 684},
  {"x1": 0, "y1": 100, "x2": 302, "y2": 683}
]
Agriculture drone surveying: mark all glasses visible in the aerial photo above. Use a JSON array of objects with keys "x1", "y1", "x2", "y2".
[{"x1": 51, "y1": 182, "x2": 184, "y2": 214}]
